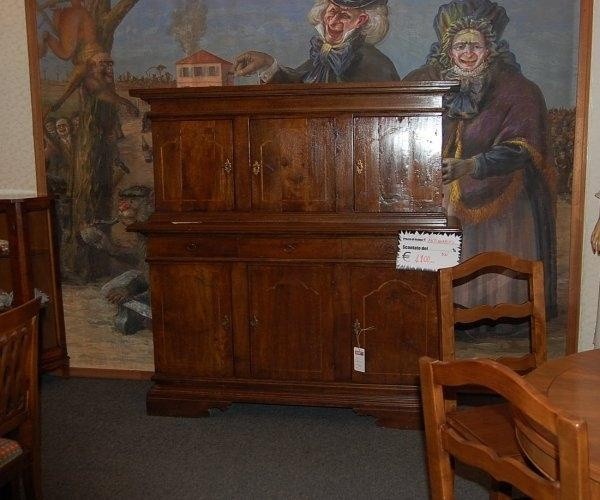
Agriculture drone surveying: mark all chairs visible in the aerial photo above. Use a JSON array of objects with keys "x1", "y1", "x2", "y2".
[
  {"x1": 416, "y1": 351, "x2": 587, "y2": 498},
  {"x1": 1, "y1": 296, "x2": 45, "y2": 500},
  {"x1": 436, "y1": 249, "x2": 550, "y2": 483}
]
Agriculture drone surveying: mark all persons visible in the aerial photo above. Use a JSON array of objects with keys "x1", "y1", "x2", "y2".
[
  {"x1": 234, "y1": 0, "x2": 401, "y2": 85},
  {"x1": 402, "y1": 0, "x2": 557, "y2": 343}
]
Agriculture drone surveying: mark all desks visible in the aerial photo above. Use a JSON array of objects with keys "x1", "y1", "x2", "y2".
[{"x1": 511, "y1": 345, "x2": 597, "y2": 495}]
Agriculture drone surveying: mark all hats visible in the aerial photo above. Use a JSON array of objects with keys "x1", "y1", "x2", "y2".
[
  {"x1": 121, "y1": 186, "x2": 151, "y2": 197},
  {"x1": 329, "y1": 0, "x2": 388, "y2": 8},
  {"x1": 42, "y1": 117, "x2": 57, "y2": 125}
]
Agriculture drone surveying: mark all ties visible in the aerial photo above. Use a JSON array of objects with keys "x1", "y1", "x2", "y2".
[{"x1": 65, "y1": 137, "x2": 71, "y2": 150}]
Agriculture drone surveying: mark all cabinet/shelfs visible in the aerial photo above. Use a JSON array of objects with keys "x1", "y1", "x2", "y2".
[{"x1": 122, "y1": 77, "x2": 463, "y2": 434}]
